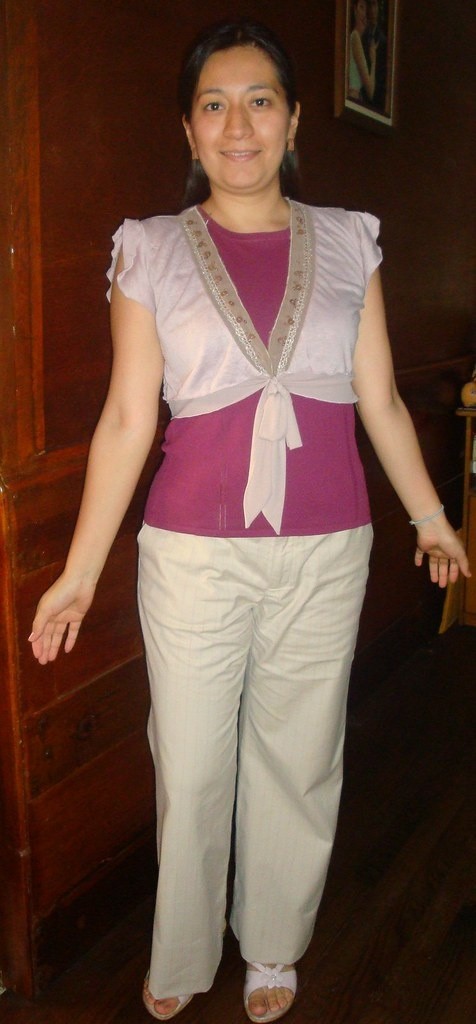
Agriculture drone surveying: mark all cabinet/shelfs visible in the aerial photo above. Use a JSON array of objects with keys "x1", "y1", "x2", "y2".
[
  {"x1": 0, "y1": 0, "x2": 213, "y2": 1005},
  {"x1": 456, "y1": 404, "x2": 476, "y2": 626}
]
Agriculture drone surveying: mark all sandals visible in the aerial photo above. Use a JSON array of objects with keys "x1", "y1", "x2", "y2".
[
  {"x1": 243, "y1": 960, "x2": 298, "y2": 1024},
  {"x1": 142, "y1": 971, "x2": 194, "y2": 1020}
]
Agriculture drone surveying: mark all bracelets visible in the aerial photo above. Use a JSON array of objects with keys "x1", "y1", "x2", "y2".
[{"x1": 409, "y1": 505, "x2": 444, "y2": 526}]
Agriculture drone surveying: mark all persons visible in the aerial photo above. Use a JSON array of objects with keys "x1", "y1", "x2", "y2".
[
  {"x1": 27, "y1": 24, "x2": 473, "y2": 1023},
  {"x1": 348, "y1": 1, "x2": 389, "y2": 106}
]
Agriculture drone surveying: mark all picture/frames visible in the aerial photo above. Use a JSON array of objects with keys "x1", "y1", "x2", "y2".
[{"x1": 333, "y1": 0, "x2": 405, "y2": 146}]
